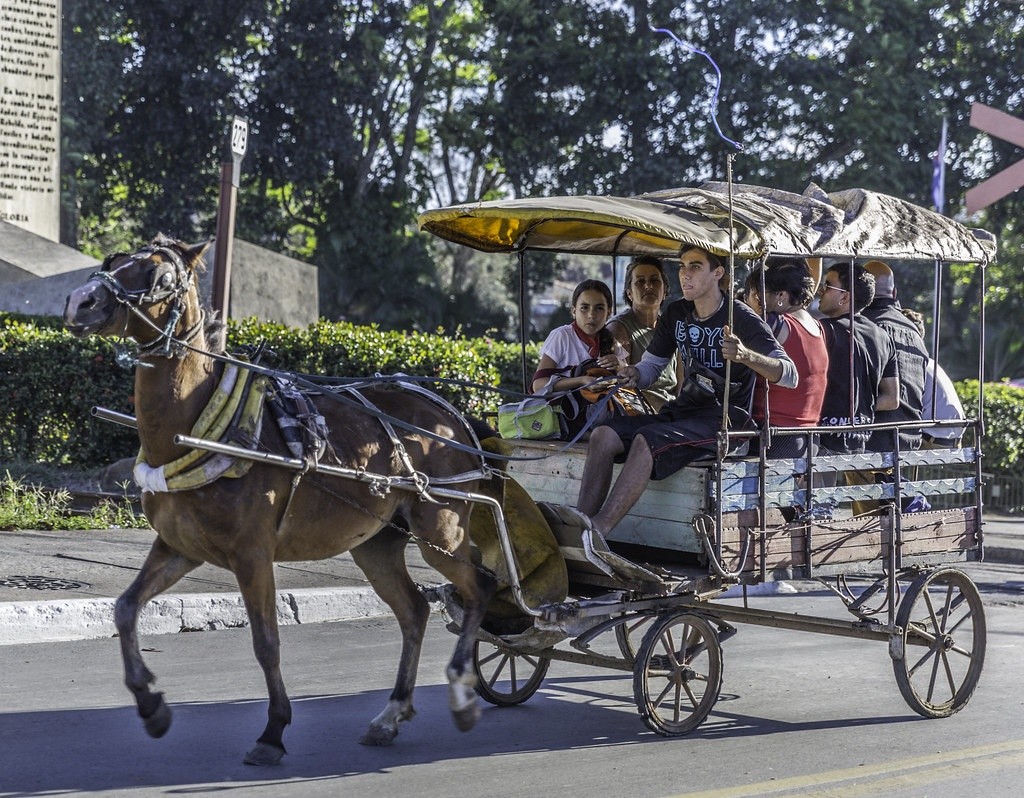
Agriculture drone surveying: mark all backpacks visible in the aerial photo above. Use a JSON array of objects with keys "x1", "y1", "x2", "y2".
[{"x1": 568, "y1": 357, "x2": 658, "y2": 430}]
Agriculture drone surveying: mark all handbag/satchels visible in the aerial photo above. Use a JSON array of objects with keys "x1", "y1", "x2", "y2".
[
  {"x1": 678, "y1": 349, "x2": 741, "y2": 410},
  {"x1": 498, "y1": 399, "x2": 563, "y2": 441}
]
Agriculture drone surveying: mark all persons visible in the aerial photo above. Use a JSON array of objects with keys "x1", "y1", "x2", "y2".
[
  {"x1": 738, "y1": 258, "x2": 823, "y2": 317},
  {"x1": 746, "y1": 257, "x2": 829, "y2": 459},
  {"x1": 875, "y1": 308, "x2": 966, "y2": 513},
  {"x1": 844, "y1": 261, "x2": 930, "y2": 517},
  {"x1": 532, "y1": 279, "x2": 630, "y2": 442},
  {"x1": 536, "y1": 243, "x2": 799, "y2": 538},
  {"x1": 606, "y1": 256, "x2": 685, "y2": 412},
  {"x1": 795, "y1": 262, "x2": 900, "y2": 520}
]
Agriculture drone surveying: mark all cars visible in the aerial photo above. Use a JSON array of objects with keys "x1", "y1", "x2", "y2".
[{"x1": 529, "y1": 299, "x2": 562, "y2": 336}]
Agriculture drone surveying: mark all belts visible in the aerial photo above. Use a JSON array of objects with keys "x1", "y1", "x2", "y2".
[{"x1": 921, "y1": 432, "x2": 962, "y2": 445}]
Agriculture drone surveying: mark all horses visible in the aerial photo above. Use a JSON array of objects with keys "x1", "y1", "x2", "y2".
[{"x1": 62, "y1": 231, "x2": 505, "y2": 768}]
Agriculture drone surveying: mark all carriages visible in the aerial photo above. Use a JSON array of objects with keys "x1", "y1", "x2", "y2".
[{"x1": 62, "y1": 177, "x2": 997, "y2": 769}]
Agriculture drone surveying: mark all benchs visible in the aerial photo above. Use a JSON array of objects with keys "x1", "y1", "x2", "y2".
[{"x1": 507, "y1": 438, "x2": 795, "y2": 572}]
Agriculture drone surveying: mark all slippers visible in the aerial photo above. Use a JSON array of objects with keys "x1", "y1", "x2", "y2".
[
  {"x1": 535, "y1": 500, "x2": 565, "y2": 526},
  {"x1": 556, "y1": 505, "x2": 593, "y2": 530}
]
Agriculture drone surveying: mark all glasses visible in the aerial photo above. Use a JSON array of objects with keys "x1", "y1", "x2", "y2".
[
  {"x1": 822, "y1": 281, "x2": 847, "y2": 293},
  {"x1": 755, "y1": 290, "x2": 774, "y2": 302}
]
